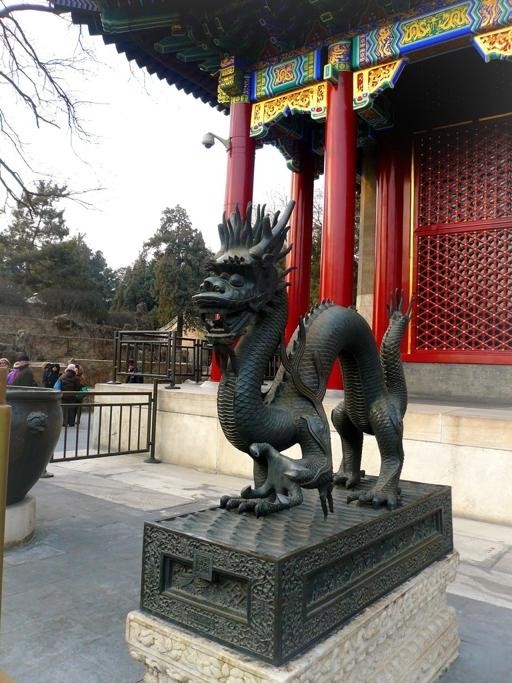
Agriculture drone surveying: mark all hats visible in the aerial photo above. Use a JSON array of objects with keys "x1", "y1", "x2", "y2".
[
  {"x1": 0, "y1": 358, "x2": 30, "y2": 368},
  {"x1": 65, "y1": 357, "x2": 78, "y2": 375}
]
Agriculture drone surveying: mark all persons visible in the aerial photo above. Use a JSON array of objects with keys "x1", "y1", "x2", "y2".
[{"x1": 1, "y1": 359, "x2": 142, "y2": 428}]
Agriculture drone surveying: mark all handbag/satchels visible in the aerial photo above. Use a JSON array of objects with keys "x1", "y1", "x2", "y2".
[
  {"x1": 75, "y1": 386, "x2": 89, "y2": 400},
  {"x1": 53, "y1": 377, "x2": 61, "y2": 390}
]
